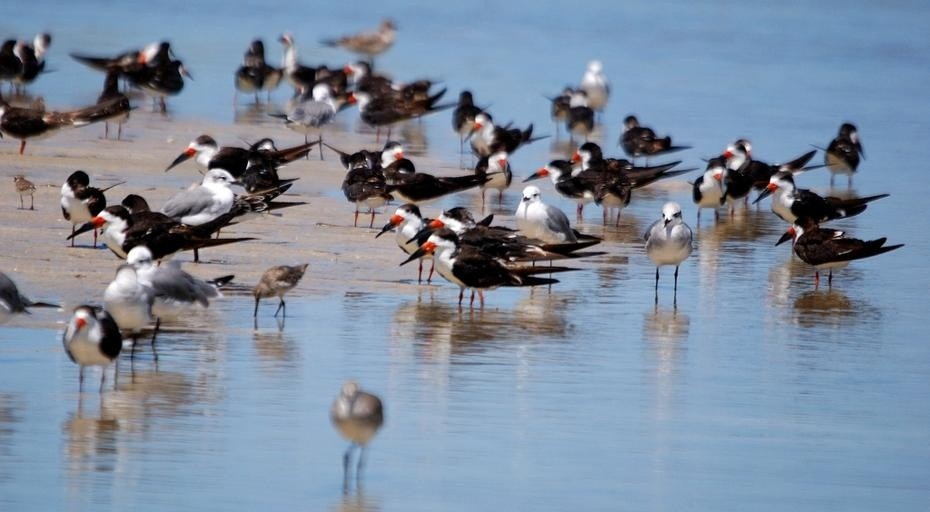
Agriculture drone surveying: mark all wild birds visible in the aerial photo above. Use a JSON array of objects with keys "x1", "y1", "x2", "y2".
[
  {"x1": 0, "y1": 18, "x2": 907, "y2": 345},
  {"x1": 328, "y1": 382, "x2": 382, "y2": 459},
  {"x1": 61, "y1": 301, "x2": 124, "y2": 395}
]
